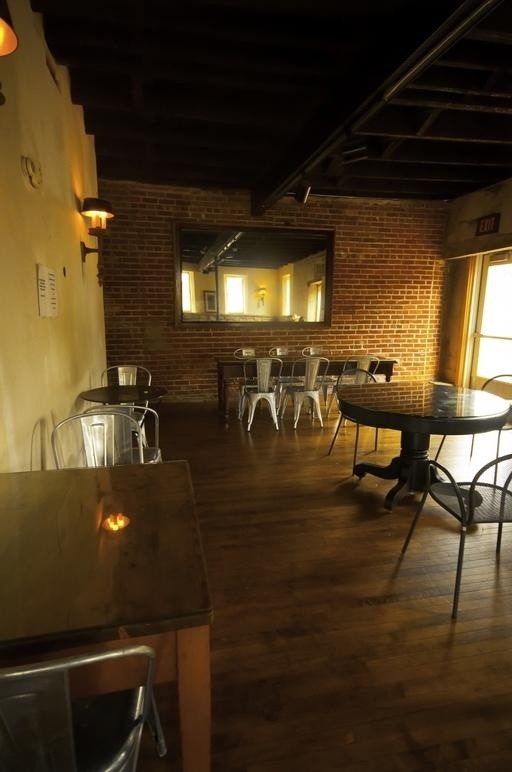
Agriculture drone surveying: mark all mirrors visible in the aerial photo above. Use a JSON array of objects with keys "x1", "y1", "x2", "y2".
[{"x1": 173, "y1": 222, "x2": 336, "y2": 329}]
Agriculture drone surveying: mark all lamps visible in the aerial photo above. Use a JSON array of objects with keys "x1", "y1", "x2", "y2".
[
  {"x1": 257, "y1": 288, "x2": 267, "y2": 300},
  {"x1": 0, "y1": 0, "x2": 19, "y2": 106},
  {"x1": 81, "y1": 197, "x2": 115, "y2": 262}
]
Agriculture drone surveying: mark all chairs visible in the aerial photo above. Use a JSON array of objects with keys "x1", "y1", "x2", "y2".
[
  {"x1": 234, "y1": 345, "x2": 380, "y2": 475},
  {"x1": 79, "y1": 404, "x2": 163, "y2": 468},
  {"x1": 435, "y1": 375, "x2": 512, "y2": 484},
  {"x1": 401, "y1": 454, "x2": 512, "y2": 619},
  {"x1": 101, "y1": 365, "x2": 152, "y2": 448},
  {"x1": 51, "y1": 411, "x2": 145, "y2": 470},
  {"x1": 0, "y1": 459, "x2": 214, "y2": 772},
  {"x1": 0, "y1": 645, "x2": 167, "y2": 772}
]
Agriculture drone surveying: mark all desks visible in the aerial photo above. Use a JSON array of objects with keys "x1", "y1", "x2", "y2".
[
  {"x1": 79, "y1": 385, "x2": 168, "y2": 451},
  {"x1": 337, "y1": 381, "x2": 511, "y2": 513}
]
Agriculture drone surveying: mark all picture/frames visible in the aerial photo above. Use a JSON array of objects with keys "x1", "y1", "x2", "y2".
[{"x1": 203, "y1": 291, "x2": 218, "y2": 313}]
